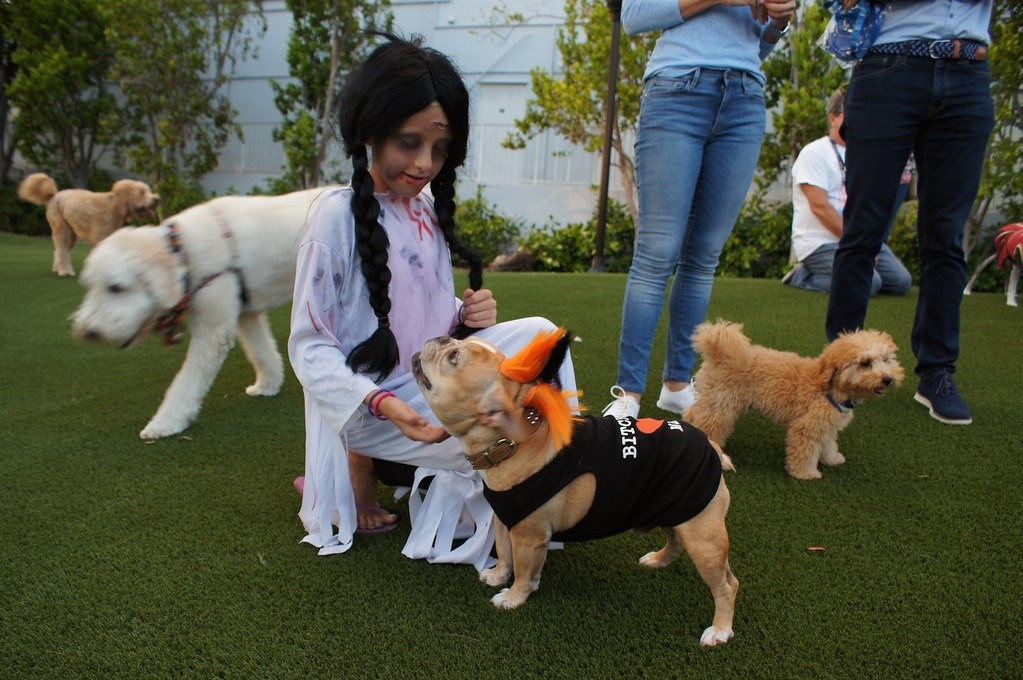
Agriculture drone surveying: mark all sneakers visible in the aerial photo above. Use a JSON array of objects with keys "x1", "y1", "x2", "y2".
[
  {"x1": 656, "y1": 375, "x2": 698, "y2": 415},
  {"x1": 601, "y1": 385, "x2": 641, "y2": 420},
  {"x1": 914, "y1": 372, "x2": 973, "y2": 425}
]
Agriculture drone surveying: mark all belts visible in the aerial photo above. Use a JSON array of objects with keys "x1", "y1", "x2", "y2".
[{"x1": 868, "y1": 38, "x2": 988, "y2": 61}]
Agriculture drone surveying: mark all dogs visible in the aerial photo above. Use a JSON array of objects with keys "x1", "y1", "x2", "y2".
[
  {"x1": 682, "y1": 318, "x2": 905, "y2": 479},
  {"x1": 70, "y1": 184, "x2": 360, "y2": 440},
  {"x1": 962, "y1": 221, "x2": 1023, "y2": 307},
  {"x1": 410, "y1": 330, "x2": 740, "y2": 649},
  {"x1": 18, "y1": 172, "x2": 159, "y2": 275}
]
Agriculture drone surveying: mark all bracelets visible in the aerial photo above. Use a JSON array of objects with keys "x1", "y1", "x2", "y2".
[
  {"x1": 374, "y1": 394, "x2": 396, "y2": 421},
  {"x1": 368, "y1": 390, "x2": 389, "y2": 417}
]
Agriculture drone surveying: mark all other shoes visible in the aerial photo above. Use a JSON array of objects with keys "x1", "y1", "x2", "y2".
[{"x1": 780, "y1": 262, "x2": 804, "y2": 285}]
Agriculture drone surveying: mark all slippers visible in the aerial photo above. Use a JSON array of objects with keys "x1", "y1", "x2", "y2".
[{"x1": 293, "y1": 474, "x2": 398, "y2": 534}]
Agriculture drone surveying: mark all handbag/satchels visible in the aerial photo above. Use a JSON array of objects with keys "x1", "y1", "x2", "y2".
[{"x1": 822, "y1": 0, "x2": 896, "y2": 60}]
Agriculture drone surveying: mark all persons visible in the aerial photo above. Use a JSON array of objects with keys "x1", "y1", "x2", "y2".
[
  {"x1": 288, "y1": 40, "x2": 580, "y2": 571},
  {"x1": 825, "y1": 0, "x2": 993, "y2": 424},
  {"x1": 781, "y1": 89, "x2": 912, "y2": 297},
  {"x1": 604, "y1": 0, "x2": 795, "y2": 419}
]
